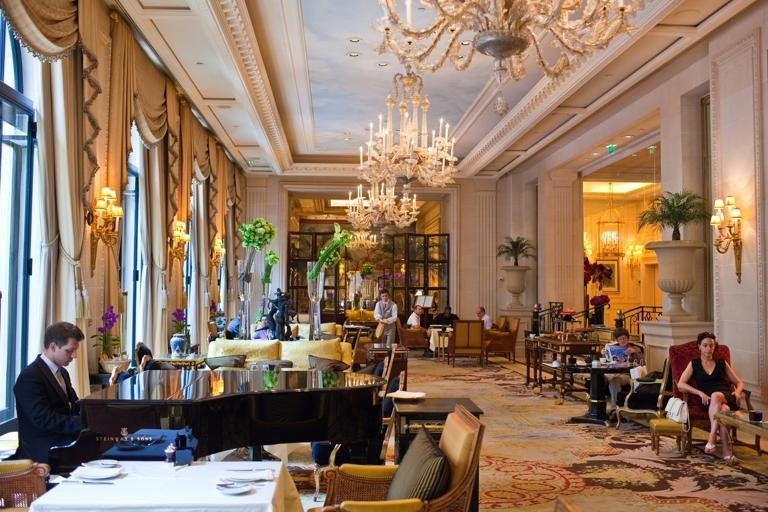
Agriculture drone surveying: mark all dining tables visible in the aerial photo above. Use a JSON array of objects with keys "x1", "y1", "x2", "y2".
[
  {"x1": 494, "y1": 234, "x2": 534, "y2": 309},
  {"x1": 638, "y1": 191, "x2": 711, "y2": 317}
]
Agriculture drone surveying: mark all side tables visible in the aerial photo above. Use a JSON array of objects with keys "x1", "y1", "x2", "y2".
[{"x1": 392, "y1": 397, "x2": 484, "y2": 511}]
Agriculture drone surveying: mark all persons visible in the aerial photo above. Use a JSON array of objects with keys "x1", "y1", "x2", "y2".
[
  {"x1": 627, "y1": 344, "x2": 670, "y2": 391},
  {"x1": 677, "y1": 333, "x2": 746, "y2": 463},
  {"x1": 13, "y1": 321, "x2": 86, "y2": 466},
  {"x1": 476, "y1": 306, "x2": 492, "y2": 330},
  {"x1": 432, "y1": 307, "x2": 459, "y2": 328},
  {"x1": 601, "y1": 330, "x2": 641, "y2": 421},
  {"x1": 406, "y1": 305, "x2": 433, "y2": 357},
  {"x1": 374, "y1": 290, "x2": 398, "y2": 348}
]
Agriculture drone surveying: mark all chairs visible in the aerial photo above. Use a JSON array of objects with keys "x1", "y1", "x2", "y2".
[
  {"x1": 309, "y1": 402, "x2": 484, "y2": 512},
  {"x1": 484, "y1": 315, "x2": 522, "y2": 363},
  {"x1": 312, "y1": 370, "x2": 406, "y2": 502},
  {"x1": 396, "y1": 317, "x2": 428, "y2": 357},
  {"x1": 109, "y1": 308, "x2": 407, "y2": 406},
  {"x1": 448, "y1": 319, "x2": 484, "y2": 365},
  {"x1": 1, "y1": 459, "x2": 51, "y2": 512},
  {"x1": 523, "y1": 327, "x2": 768, "y2": 464}
]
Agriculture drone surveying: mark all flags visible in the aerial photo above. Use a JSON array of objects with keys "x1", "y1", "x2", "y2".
[
  {"x1": 239, "y1": 242, "x2": 256, "y2": 283},
  {"x1": 583, "y1": 283, "x2": 588, "y2": 294},
  {"x1": 262, "y1": 261, "x2": 273, "y2": 284},
  {"x1": 594, "y1": 306, "x2": 605, "y2": 324}
]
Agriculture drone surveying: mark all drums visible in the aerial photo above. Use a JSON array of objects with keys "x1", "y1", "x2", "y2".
[
  {"x1": 62, "y1": 479, "x2": 114, "y2": 486},
  {"x1": 224, "y1": 478, "x2": 274, "y2": 483},
  {"x1": 227, "y1": 468, "x2": 275, "y2": 471},
  {"x1": 148, "y1": 435, "x2": 163, "y2": 446}
]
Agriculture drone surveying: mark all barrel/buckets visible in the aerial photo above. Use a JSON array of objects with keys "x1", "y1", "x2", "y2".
[{"x1": 704, "y1": 442, "x2": 736, "y2": 461}]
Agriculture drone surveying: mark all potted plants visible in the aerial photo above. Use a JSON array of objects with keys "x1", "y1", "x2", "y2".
[
  {"x1": 594, "y1": 257, "x2": 620, "y2": 293},
  {"x1": 494, "y1": 234, "x2": 534, "y2": 309},
  {"x1": 638, "y1": 191, "x2": 711, "y2": 317}
]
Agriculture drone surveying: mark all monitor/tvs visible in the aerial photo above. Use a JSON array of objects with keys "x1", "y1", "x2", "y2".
[
  {"x1": 605, "y1": 345, "x2": 626, "y2": 362},
  {"x1": 415, "y1": 295, "x2": 433, "y2": 307},
  {"x1": 629, "y1": 365, "x2": 648, "y2": 390}
]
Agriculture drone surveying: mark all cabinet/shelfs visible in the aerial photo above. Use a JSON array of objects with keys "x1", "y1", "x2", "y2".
[{"x1": 364, "y1": 342, "x2": 409, "y2": 418}]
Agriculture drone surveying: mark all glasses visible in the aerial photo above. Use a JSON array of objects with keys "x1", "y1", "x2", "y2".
[
  {"x1": 353, "y1": 3, "x2": 462, "y2": 189},
  {"x1": 209, "y1": 235, "x2": 227, "y2": 283},
  {"x1": 372, "y1": 1, "x2": 646, "y2": 124},
  {"x1": 344, "y1": 173, "x2": 427, "y2": 230},
  {"x1": 710, "y1": 196, "x2": 745, "y2": 282},
  {"x1": 165, "y1": 217, "x2": 192, "y2": 284},
  {"x1": 86, "y1": 187, "x2": 126, "y2": 249}
]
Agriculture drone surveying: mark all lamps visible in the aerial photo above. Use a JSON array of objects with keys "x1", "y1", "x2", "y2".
[
  {"x1": 372, "y1": 1, "x2": 646, "y2": 124},
  {"x1": 86, "y1": 187, "x2": 126, "y2": 249},
  {"x1": 344, "y1": 173, "x2": 427, "y2": 230},
  {"x1": 710, "y1": 196, "x2": 745, "y2": 282},
  {"x1": 353, "y1": 3, "x2": 462, "y2": 189},
  {"x1": 165, "y1": 217, "x2": 192, "y2": 284},
  {"x1": 523, "y1": 327, "x2": 768, "y2": 464},
  {"x1": 209, "y1": 235, "x2": 227, "y2": 283}
]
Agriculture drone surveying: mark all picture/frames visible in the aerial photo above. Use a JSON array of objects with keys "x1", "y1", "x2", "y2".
[{"x1": 594, "y1": 257, "x2": 620, "y2": 293}]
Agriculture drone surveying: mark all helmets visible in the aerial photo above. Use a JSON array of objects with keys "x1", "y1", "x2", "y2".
[
  {"x1": 386, "y1": 389, "x2": 427, "y2": 400},
  {"x1": 117, "y1": 441, "x2": 145, "y2": 450},
  {"x1": 73, "y1": 458, "x2": 121, "y2": 481},
  {"x1": 214, "y1": 480, "x2": 253, "y2": 496}
]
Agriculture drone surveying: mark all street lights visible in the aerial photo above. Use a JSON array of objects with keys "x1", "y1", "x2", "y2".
[
  {"x1": 630, "y1": 359, "x2": 648, "y2": 393},
  {"x1": 664, "y1": 396, "x2": 691, "y2": 433}
]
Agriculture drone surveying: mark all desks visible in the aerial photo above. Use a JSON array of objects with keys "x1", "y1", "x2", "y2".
[
  {"x1": 30, "y1": 461, "x2": 284, "y2": 512},
  {"x1": 428, "y1": 324, "x2": 456, "y2": 361}
]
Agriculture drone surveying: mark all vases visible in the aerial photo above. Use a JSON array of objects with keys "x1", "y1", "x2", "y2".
[
  {"x1": 264, "y1": 248, "x2": 278, "y2": 284},
  {"x1": 590, "y1": 294, "x2": 610, "y2": 306},
  {"x1": 239, "y1": 215, "x2": 277, "y2": 279},
  {"x1": 583, "y1": 256, "x2": 613, "y2": 294},
  {"x1": 262, "y1": 261, "x2": 273, "y2": 284},
  {"x1": 594, "y1": 306, "x2": 605, "y2": 324},
  {"x1": 583, "y1": 283, "x2": 588, "y2": 294},
  {"x1": 239, "y1": 242, "x2": 256, "y2": 283}
]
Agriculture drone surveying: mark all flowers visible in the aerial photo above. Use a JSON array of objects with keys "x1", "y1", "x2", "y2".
[
  {"x1": 583, "y1": 256, "x2": 613, "y2": 294},
  {"x1": 239, "y1": 215, "x2": 277, "y2": 279},
  {"x1": 590, "y1": 294, "x2": 610, "y2": 306},
  {"x1": 264, "y1": 248, "x2": 278, "y2": 284}
]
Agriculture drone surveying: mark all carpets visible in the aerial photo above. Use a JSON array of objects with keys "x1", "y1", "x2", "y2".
[{"x1": 56, "y1": 368, "x2": 67, "y2": 394}]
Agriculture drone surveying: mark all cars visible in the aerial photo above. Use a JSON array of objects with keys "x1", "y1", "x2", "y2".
[
  {"x1": 600, "y1": 357, "x2": 607, "y2": 362},
  {"x1": 530, "y1": 333, "x2": 536, "y2": 339},
  {"x1": 749, "y1": 410, "x2": 763, "y2": 422}
]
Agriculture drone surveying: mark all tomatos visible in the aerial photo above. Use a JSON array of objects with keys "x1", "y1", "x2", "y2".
[{"x1": 50, "y1": 370, "x2": 386, "y2": 460}]
